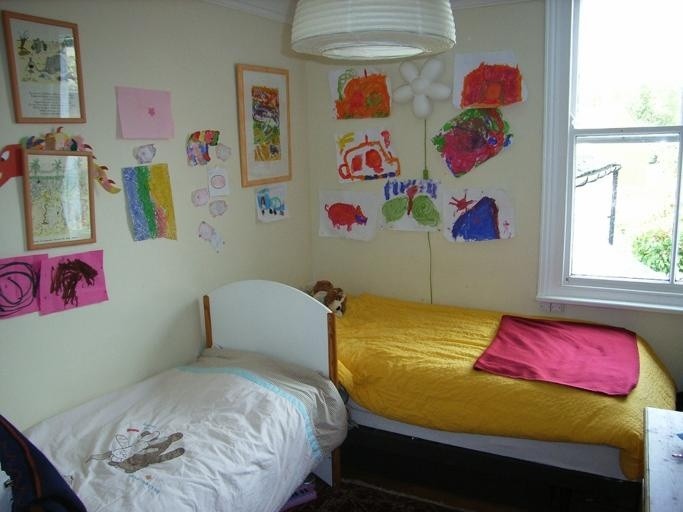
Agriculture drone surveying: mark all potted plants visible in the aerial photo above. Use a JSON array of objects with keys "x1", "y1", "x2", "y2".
[
  {"x1": 0, "y1": 9, "x2": 86, "y2": 123},
  {"x1": 21, "y1": 149, "x2": 96, "y2": 251},
  {"x1": 235, "y1": 62, "x2": 292, "y2": 188}
]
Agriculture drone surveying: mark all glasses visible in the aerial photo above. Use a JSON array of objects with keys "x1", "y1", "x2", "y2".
[{"x1": 290, "y1": 0, "x2": 458, "y2": 63}]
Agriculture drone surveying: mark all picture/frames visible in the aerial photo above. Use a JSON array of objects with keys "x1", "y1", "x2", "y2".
[{"x1": 642, "y1": 405, "x2": 683, "y2": 512}]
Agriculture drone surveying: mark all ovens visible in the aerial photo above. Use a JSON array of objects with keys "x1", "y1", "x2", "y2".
[
  {"x1": 309, "y1": 275, "x2": 677, "y2": 483},
  {"x1": 1, "y1": 280, "x2": 350, "y2": 512}
]
